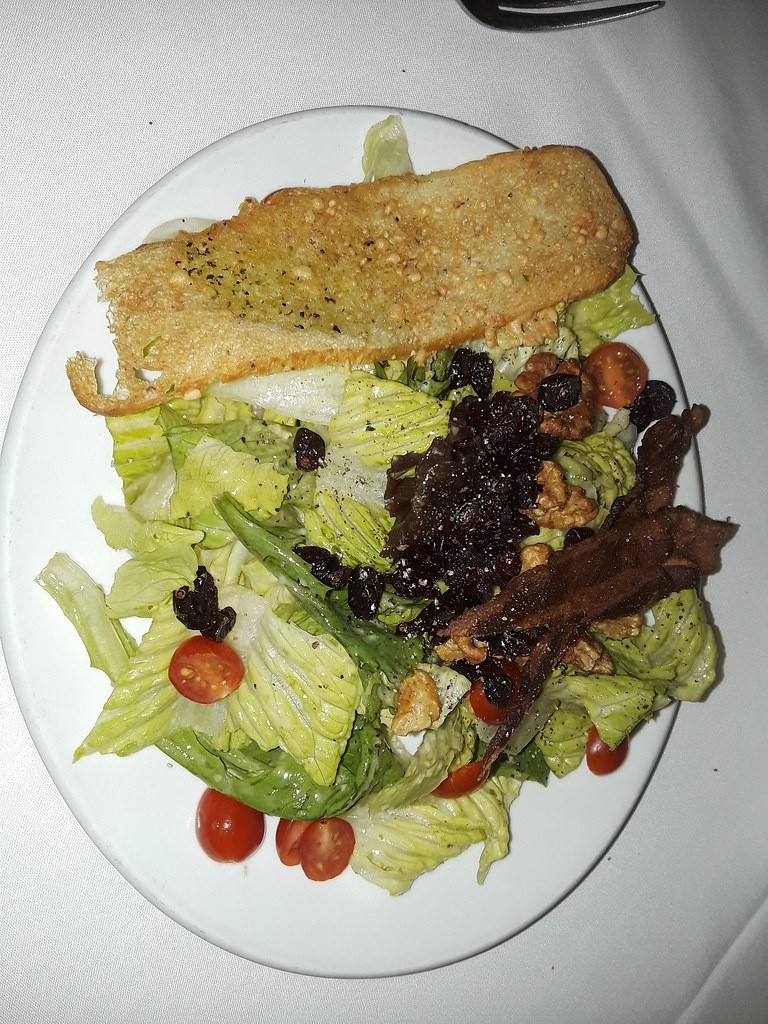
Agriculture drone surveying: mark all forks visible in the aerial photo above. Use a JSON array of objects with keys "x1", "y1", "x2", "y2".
[{"x1": 457, "y1": 0, "x2": 663, "y2": 32}]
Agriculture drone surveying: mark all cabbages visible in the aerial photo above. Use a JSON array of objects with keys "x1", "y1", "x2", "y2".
[{"x1": 36, "y1": 113, "x2": 722, "y2": 893}]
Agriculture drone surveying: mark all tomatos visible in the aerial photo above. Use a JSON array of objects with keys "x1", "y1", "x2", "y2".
[{"x1": 168, "y1": 341, "x2": 649, "y2": 880}]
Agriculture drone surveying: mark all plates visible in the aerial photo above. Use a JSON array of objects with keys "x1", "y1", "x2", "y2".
[{"x1": 0, "y1": 103, "x2": 705, "y2": 977}]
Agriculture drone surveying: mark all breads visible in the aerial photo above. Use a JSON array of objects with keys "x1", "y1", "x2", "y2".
[{"x1": 64, "y1": 145, "x2": 638, "y2": 415}]
